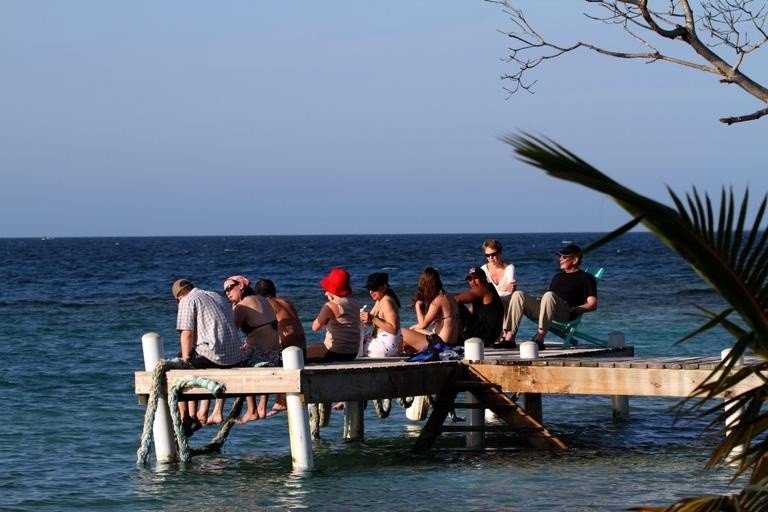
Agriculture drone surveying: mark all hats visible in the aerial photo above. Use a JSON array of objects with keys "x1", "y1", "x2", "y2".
[
  {"x1": 465, "y1": 268, "x2": 485, "y2": 280},
  {"x1": 172, "y1": 279, "x2": 192, "y2": 304},
  {"x1": 364, "y1": 273, "x2": 388, "y2": 290},
  {"x1": 320, "y1": 268, "x2": 352, "y2": 297},
  {"x1": 555, "y1": 245, "x2": 582, "y2": 258}
]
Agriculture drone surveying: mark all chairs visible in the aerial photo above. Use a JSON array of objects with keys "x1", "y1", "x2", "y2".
[{"x1": 527, "y1": 266, "x2": 614, "y2": 351}]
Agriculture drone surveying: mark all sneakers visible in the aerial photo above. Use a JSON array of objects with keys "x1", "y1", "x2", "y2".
[
  {"x1": 532, "y1": 338, "x2": 545, "y2": 350},
  {"x1": 490, "y1": 340, "x2": 516, "y2": 349},
  {"x1": 183, "y1": 416, "x2": 202, "y2": 436}
]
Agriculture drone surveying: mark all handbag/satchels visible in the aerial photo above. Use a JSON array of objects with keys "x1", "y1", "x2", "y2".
[{"x1": 405, "y1": 334, "x2": 463, "y2": 362}]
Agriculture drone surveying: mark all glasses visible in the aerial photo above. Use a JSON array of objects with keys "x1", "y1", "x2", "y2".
[
  {"x1": 224, "y1": 282, "x2": 239, "y2": 295},
  {"x1": 485, "y1": 250, "x2": 500, "y2": 257}
]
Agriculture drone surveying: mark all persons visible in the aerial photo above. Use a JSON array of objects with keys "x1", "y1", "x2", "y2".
[
  {"x1": 491, "y1": 245, "x2": 595, "y2": 348},
  {"x1": 267, "y1": 268, "x2": 360, "y2": 417},
  {"x1": 453, "y1": 240, "x2": 516, "y2": 346},
  {"x1": 332, "y1": 272, "x2": 404, "y2": 411},
  {"x1": 172, "y1": 274, "x2": 305, "y2": 425},
  {"x1": 401, "y1": 267, "x2": 459, "y2": 351}
]
